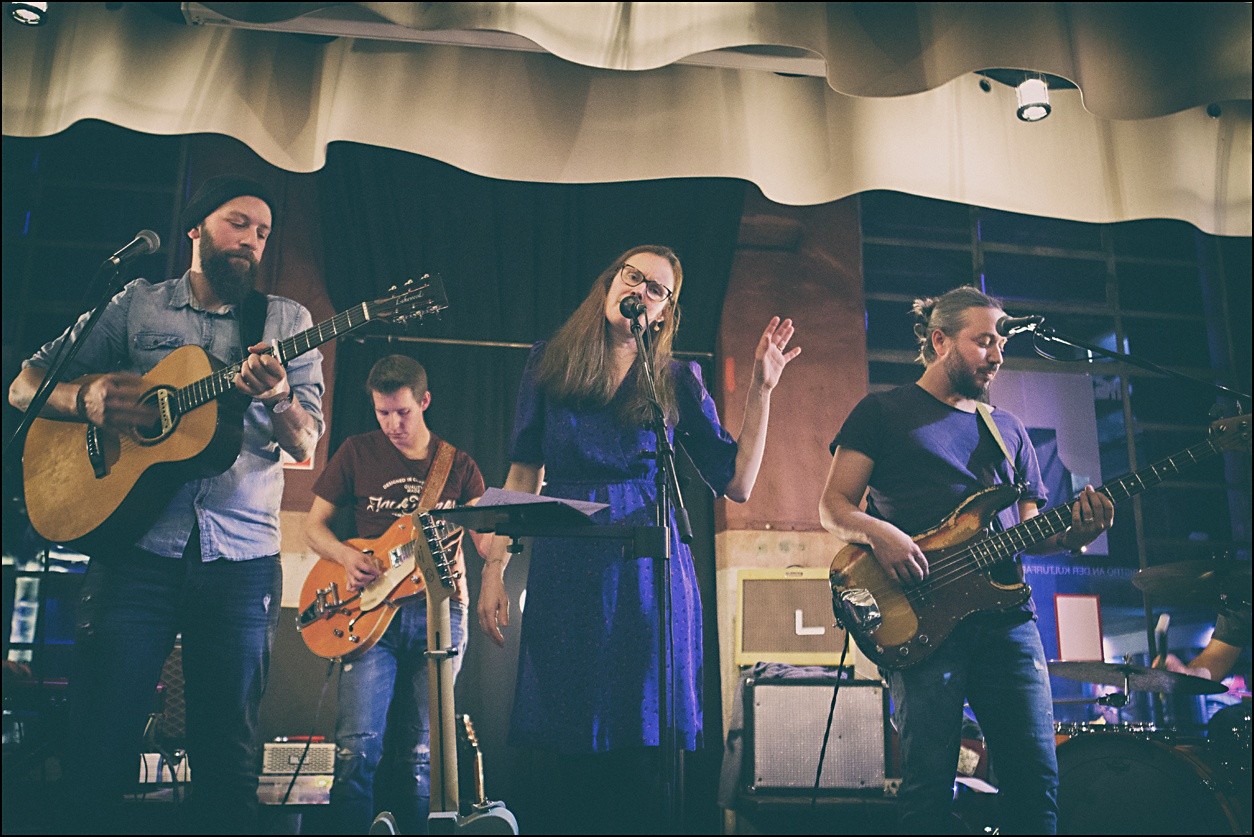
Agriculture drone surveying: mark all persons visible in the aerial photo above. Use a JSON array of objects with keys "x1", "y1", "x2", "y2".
[
  {"x1": 819, "y1": 286, "x2": 1115, "y2": 837},
  {"x1": 7, "y1": 170, "x2": 326, "y2": 837},
  {"x1": 304, "y1": 356, "x2": 497, "y2": 837},
  {"x1": 477, "y1": 246, "x2": 800, "y2": 837},
  {"x1": 1150, "y1": 555, "x2": 1253, "y2": 696}
]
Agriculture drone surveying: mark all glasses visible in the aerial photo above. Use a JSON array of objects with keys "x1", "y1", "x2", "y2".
[{"x1": 620, "y1": 262, "x2": 674, "y2": 303}]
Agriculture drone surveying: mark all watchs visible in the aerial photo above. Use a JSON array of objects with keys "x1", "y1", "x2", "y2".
[{"x1": 261, "y1": 386, "x2": 294, "y2": 414}]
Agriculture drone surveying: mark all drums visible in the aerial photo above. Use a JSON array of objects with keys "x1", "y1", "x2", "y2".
[{"x1": 1055, "y1": 731, "x2": 1246, "y2": 835}]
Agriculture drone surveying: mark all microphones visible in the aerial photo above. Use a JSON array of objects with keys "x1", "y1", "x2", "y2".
[
  {"x1": 994, "y1": 313, "x2": 1043, "y2": 338},
  {"x1": 95, "y1": 227, "x2": 163, "y2": 269},
  {"x1": 617, "y1": 293, "x2": 650, "y2": 319}
]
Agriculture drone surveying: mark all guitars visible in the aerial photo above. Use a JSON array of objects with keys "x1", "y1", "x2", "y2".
[
  {"x1": 297, "y1": 514, "x2": 464, "y2": 665},
  {"x1": 829, "y1": 413, "x2": 1252, "y2": 673},
  {"x1": 23, "y1": 272, "x2": 449, "y2": 556},
  {"x1": 369, "y1": 507, "x2": 519, "y2": 837}
]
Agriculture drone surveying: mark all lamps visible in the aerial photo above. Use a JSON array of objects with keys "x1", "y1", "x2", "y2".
[
  {"x1": 11, "y1": 2, "x2": 48, "y2": 25},
  {"x1": 1014, "y1": 73, "x2": 1052, "y2": 122}
]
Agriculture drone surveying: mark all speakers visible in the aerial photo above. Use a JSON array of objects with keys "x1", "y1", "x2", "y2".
[{"x1": 744, "y1": 677, "x2": 891, "y2": 796}]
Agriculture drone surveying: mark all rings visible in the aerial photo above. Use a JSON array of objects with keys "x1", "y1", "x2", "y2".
[{"x1": 1085, "y1": 518, "x2": 1095, "y2": 523}]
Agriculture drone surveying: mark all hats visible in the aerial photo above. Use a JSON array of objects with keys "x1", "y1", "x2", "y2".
[{"x1": 182, "y1": 174, "x2": 277, "y2": 241}]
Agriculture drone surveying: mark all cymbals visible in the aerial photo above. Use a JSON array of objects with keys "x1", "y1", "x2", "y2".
[
  {"x1": 1047, "y1": 659, "x2": 1229, "y2": 696},
  {"x1": 1131, "y1": 559, "x2": 1252, "y2": 612}
]
